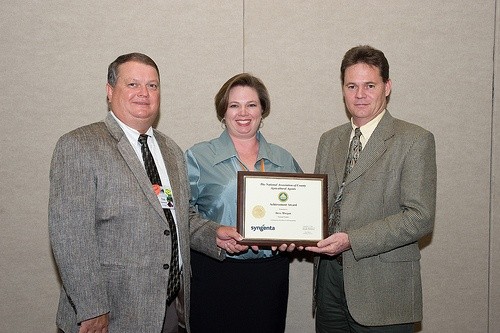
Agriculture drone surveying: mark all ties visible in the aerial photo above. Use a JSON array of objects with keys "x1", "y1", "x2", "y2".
[
  {"x1": 138, "y1": 133, "x2": 181, "y2": 305},
  {"x1": 328, "y1": 128, "x2": 363, "y2": 236}
]
